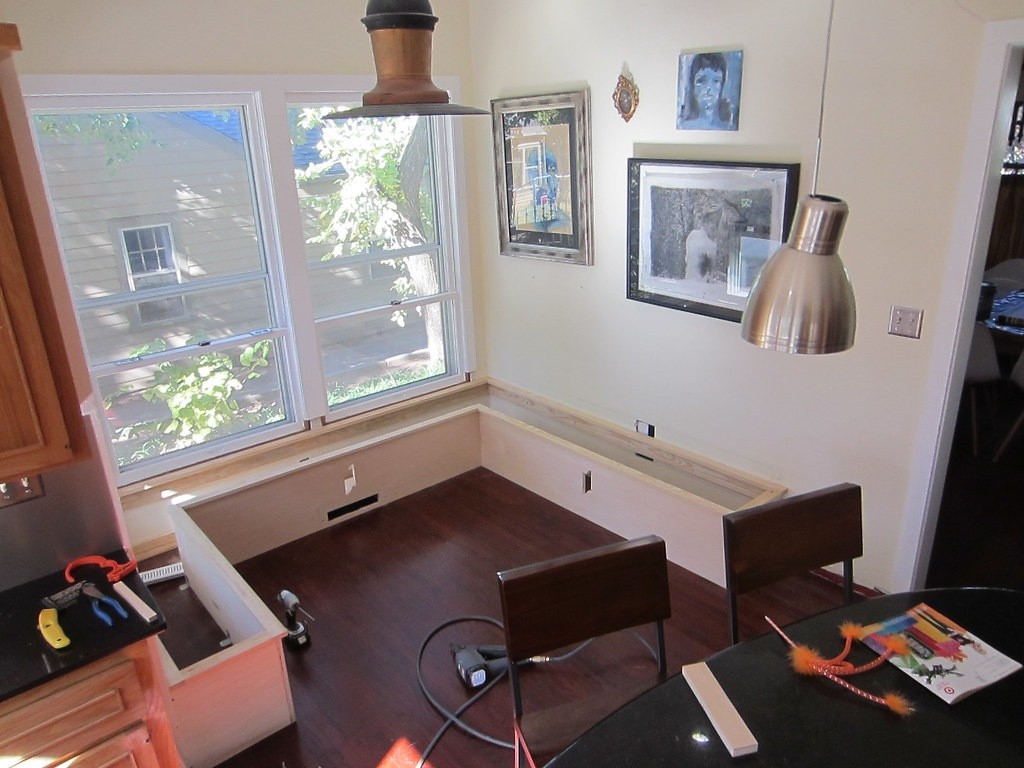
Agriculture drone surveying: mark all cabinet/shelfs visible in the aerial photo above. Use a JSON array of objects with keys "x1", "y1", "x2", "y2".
[{"x1": 0, "y1": 111, "x2": 185, "y2": 768}]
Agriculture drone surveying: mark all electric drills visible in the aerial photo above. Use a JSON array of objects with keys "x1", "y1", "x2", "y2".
[
  {"x1": 448, "y1": 641, "x2": 529, "y2": 687},
  {"x1": 277, "y1": 589, "x2": 316, "y2": 651}
]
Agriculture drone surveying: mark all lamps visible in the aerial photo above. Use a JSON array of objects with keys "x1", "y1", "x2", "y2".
[
  {"x1": 321, "y1": 0, "x2": 492, "y2": 130},
  {"x1": 739, "y1": 0, "x2": 858, "y2": 356}
]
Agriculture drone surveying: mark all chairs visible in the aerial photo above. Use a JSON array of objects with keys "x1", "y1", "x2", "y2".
[
  {"x1": 493, "y1": 535, "x2": 672, "y2": 768},
  {"x1": 721, "y1": 482, "x2": 864, "y2": 644}
]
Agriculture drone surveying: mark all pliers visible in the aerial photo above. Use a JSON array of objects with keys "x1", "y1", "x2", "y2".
[{"x1": 82, "y1": 582, "x2": 128, "y2": 627}]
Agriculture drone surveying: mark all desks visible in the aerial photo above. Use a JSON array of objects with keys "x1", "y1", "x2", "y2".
[{"x1": 545, "y1": 587, "x2": 1024, "y2": 768}]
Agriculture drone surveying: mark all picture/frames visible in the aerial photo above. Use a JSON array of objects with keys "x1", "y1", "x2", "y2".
[
  {"x1": 488, "y1": 87, "x2": 596, "y2": 267},
  {"x1": 625, "y1": 158, "x2": 802, "y2": 322}
]
